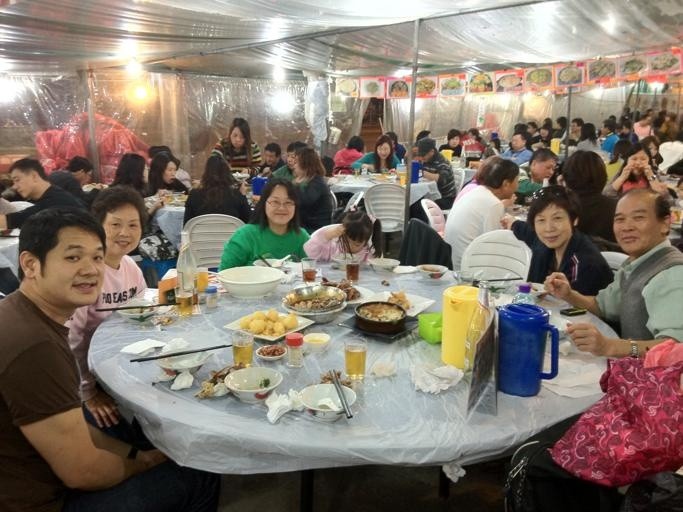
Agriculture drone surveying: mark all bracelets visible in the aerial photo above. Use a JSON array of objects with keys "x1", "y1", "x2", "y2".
[
  {"x1": 628, "y1": 337, "x2": 638, "y2": 359},
  {"x1": 126, "y1": 445, "x2": 138, "y2": 461}
]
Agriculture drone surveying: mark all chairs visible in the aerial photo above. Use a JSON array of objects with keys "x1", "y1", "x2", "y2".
[{"x1": 10, "y1": 201, "x2": 35, "y2": 236}]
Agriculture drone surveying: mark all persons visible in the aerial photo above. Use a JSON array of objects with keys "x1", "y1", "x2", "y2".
[
  {"x1": 0, "y1": 206, "x2": 225, "y2": 511},
  {"x1": 498, "y1": 183, "x2": 620, "y2": 338},
  {"x1": 67, "y1": 183, "x2": 158, "y2": 454},
  {"x1": 0, "y1": 103, "x2": 683, "y2": 270},
  {"x1": 539, "y1": 187, "x2": 682, "y2": 366}
]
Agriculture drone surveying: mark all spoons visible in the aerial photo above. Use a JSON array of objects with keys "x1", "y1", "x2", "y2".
[{"x1": 318, "y1": 397, "x2": 340, "y2": 414}]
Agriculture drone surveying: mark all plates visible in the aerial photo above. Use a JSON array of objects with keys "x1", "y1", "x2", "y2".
[{"x1": 255, "y1": 345, "x2": 287, "y2": 361}]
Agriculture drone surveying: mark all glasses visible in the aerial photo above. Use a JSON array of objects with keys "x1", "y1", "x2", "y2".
[{"x1": 266, "y1": 200, "x2": 294, "y2": 207}]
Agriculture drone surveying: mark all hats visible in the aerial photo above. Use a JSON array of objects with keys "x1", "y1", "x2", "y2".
[{"x1": 416, "y1": 138, "x2": 436, "y2": 156}]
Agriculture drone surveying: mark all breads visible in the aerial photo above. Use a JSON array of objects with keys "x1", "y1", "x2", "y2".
[{"x1": 240, "y1": 307, "x2": 297, "y2": 338}]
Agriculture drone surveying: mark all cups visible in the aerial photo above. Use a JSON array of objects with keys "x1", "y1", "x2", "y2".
[
  {"x1": 228, "y1": 328, "x2": 255, "y2": 367},
  {"x1": 331, "y1": 164, "x2": 408, "y2": 187},
  {"x1": 669, "y1": 198, "x2": 683, "y2": 225},
  {"x1": 342, "y1": 333, "x2": 369, "y2": 380},
  {"x1": 164, "y1": 191, "x2": 174, "y2": 205}
]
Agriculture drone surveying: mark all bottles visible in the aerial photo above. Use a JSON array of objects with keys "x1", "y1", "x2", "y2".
[
  {"x1": 512, "y1": 283, "x2": 538, "y2": 307},
  {"x1": 175, "y1": 230, "x2": 217, "y2": 318},
  {"x1": 464, "y1": 278, "x2": 491, "y2": 385},
  {"x1": 284, "y1": 330, "x2": 304, "y2": 369}
]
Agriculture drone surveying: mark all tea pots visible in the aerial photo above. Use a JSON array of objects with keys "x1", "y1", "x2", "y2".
[
  {"x1": 441, "y1": 283, "x2": 491, "y2": 369},
  {"x1": 441, "y1": 150, "x2": 454, "y2": 163},
  {"x1": 550, "y1": 138, "x2": 561, "y2": 157},
  {"x1": 410, "y1": 160, "x2": 423, "y2": 184}
]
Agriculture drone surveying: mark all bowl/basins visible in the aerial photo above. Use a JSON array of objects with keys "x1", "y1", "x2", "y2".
[
  {"x1": 114, "y1": 302, "x2": 160, "y2": 322},
  {"x1": 223, "y1": 365, "x2": 284, "y2": 406},
  {"x1": 215, "y1": 253, "x2": 450, "y2": 352},
  {"x1": 157, "y1": 345, "x2": 209, "y2": 376},
  {"x1": 233, "y1": 173, "x2": 250, "y2": 182},
  {"x1": 527, "y1": 282, "x2": 550, "y2": 301},
  {"x1": 492, "y1": 283, "x2": 512, "y2": 294},
  {"x1": 547, "y1": 315, "x2": 571, "y2": 341},
  {"x1": 296, "y1": 383, "x2": 358, "y2": 424}
]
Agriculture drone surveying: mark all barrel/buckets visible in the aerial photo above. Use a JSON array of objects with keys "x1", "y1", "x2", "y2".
[
  {"x1": 252, "y1": 176, "x2": 269, "y2": 194},
  {"x1": 439, "y1": 285, "x2": 485, "y2": 368},
  {"x1": 412, "y1": 161, "x2": 423, "y2": 181}
]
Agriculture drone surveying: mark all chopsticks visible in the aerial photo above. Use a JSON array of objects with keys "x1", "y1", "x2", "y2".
[
  {"x1": 326, "y1": 368, "x2": 354, "y2": 421},
  {"x1": 487, "y1": 278, "x2": 523, "y2": 282},
  {"x1": 94, "y1": 301, "x2": 182, "y2": 312},
  {"x1": 130, "y1": 342, "x2": 231, "y2": 364}
]
